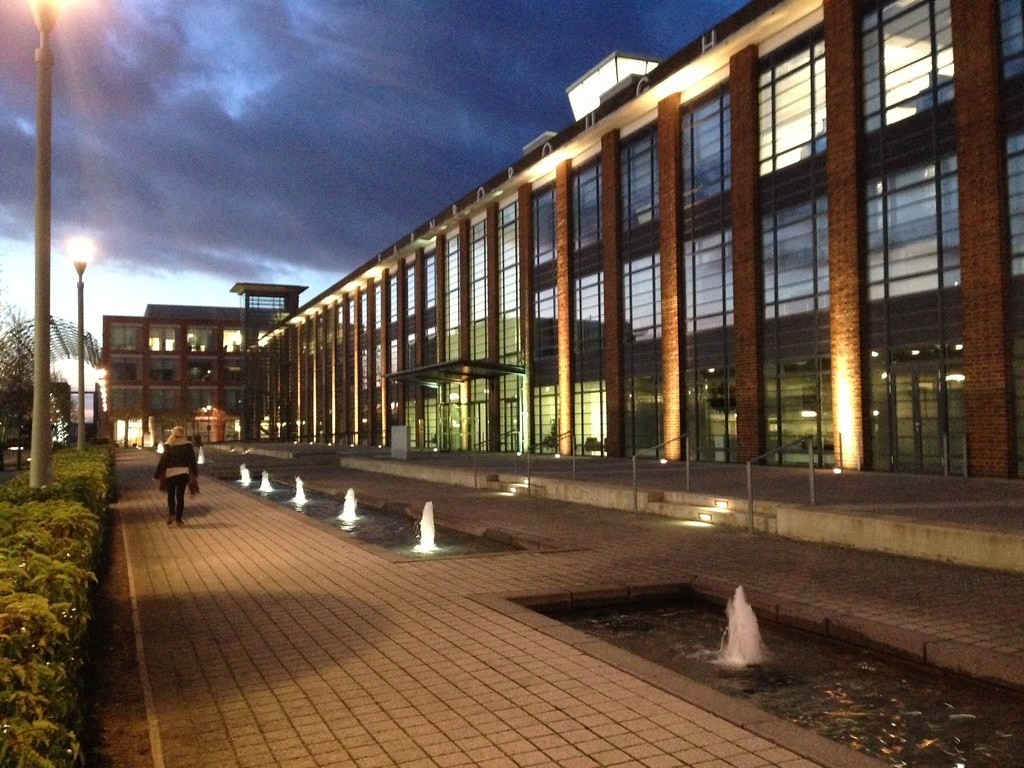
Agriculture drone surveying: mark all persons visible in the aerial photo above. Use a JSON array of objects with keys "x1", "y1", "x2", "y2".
[{"x1": 153, "y1": 426, "x2": 199, "y2": 526}]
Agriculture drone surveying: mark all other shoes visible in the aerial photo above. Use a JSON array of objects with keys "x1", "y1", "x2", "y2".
[
  {"x1": 167, "y1": 516, "x2": 174, "y2": 524},
  {"x1": 177, "y1": 521, "x2": 183, "y2": 526}
]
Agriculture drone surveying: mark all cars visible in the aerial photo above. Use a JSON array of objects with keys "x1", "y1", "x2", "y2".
[
  {"x1": 0, "y1": 442, "x2": 31, "y2": 470},
  {"x1": 89, "y1": 436, "x2": 112, "y2": 447}
]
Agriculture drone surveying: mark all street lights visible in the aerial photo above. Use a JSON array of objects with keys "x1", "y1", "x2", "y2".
[
  {"x1": 206, "y1": 405, "x2": 211, "y2": 443},
  {"x1": 29, "y1": 0, "x2": 70, "y2": 488},
  {"x1": 70, "y1": 237, "x2": 92, "y2": 451}
]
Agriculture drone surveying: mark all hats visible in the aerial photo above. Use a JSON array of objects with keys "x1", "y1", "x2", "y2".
[{"x1": 172, "y1": 426, "x2": 184, "y2": 437}]
